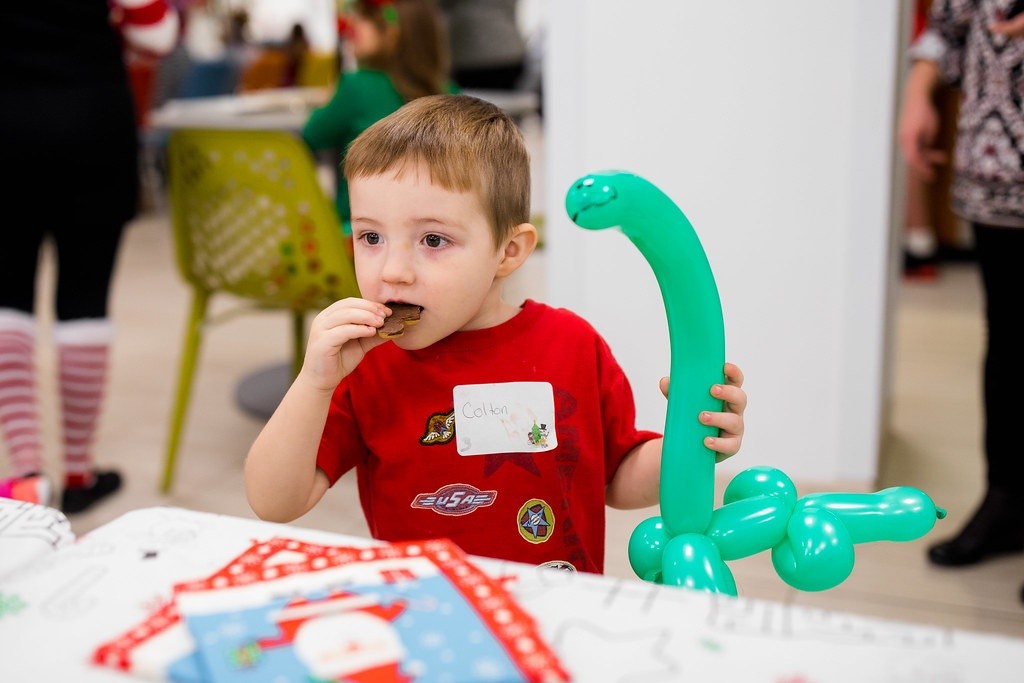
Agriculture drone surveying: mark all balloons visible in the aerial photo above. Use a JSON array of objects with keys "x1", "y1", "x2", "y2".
[{"x1": 562, "y1": 170, "x2": 948, "y2": 598}]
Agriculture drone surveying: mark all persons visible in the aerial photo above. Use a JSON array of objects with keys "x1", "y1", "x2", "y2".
[
  {"x1": 247, "y1": 95, "x2": 747, "y2": 575},
  {"x1": 892, "y1": 0, "x2": 1023, "y2": 569},
  {"x1": 0, "y1": 0, "x2": 460, "y2": 517}
]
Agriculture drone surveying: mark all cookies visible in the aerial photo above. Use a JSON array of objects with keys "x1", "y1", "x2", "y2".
[{"x1": 377, "y1": 304, "x2": 421, "y2": 338}]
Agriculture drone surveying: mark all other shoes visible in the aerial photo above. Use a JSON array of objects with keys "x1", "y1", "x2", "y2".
[
  {"x1": 928, "y1": 496, "x2": 1024, "y2": 566},
  {"x1": 938, "y1": 235, "x2": 978, "y2": 267},
  {"x1": 62, "y1": 471, "x2": 119, "y2": 514},
  {"x1": 901, "y1": 241, "x2": 938, "y2": 280}
]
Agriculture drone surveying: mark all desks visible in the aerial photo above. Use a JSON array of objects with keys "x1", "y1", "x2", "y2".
[{"x1": 0, "y1": 508, "x2": 1024, "y2": 683}]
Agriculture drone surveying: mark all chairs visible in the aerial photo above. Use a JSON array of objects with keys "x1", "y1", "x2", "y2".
[{"x1": 149, "y1": 120, "x2": 360, "y2": 502}]
